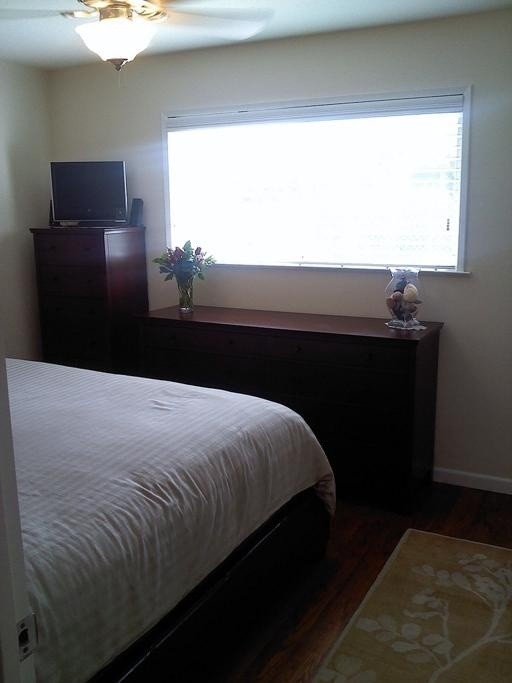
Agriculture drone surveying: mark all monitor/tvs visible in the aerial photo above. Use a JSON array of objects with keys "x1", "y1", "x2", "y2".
[{"x1": 50, "y1": 160, "x2": 127, "y2": 227}]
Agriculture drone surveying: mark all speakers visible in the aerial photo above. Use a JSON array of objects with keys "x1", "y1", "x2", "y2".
[
  {"x1": 49, "y1": 199, "x2": 60, "y2": 227},
  {"x1": 130, "y1": 198, "x2": 143, "y2": 227}
]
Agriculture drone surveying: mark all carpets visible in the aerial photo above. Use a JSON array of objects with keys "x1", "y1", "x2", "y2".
[{"x1": 315, "y1": 527, "x2": 511, "y2": 681}]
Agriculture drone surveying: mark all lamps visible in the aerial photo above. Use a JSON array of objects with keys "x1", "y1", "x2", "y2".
[{"x1": 75, "y1": 16, "x2": 161, "y2": 70}]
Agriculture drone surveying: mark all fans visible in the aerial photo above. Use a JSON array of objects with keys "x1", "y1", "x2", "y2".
[{"x1": 0, "y1": 0, "x2": 264, "y2": 45}]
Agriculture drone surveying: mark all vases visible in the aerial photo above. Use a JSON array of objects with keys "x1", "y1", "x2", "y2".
[{"x1": 174, "y1": 275, "x2": 194, "y2": 313}]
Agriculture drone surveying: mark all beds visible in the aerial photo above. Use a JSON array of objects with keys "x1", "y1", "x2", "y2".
[{"x1": 2, "y1": 344, "x2": 336, "y2": 682}]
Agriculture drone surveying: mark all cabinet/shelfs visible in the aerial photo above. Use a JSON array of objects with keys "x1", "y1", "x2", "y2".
[
  {"x1": 142, "y1": 299, "x2": 445, "y2": 515},
  {"x1": 27, "y1": 226, "x2": 151, "y2": 376}
]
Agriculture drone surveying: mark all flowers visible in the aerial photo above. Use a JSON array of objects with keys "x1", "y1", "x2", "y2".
[{"x1": 151, "y1": 237, "x2": 217, "y2": 308}]
